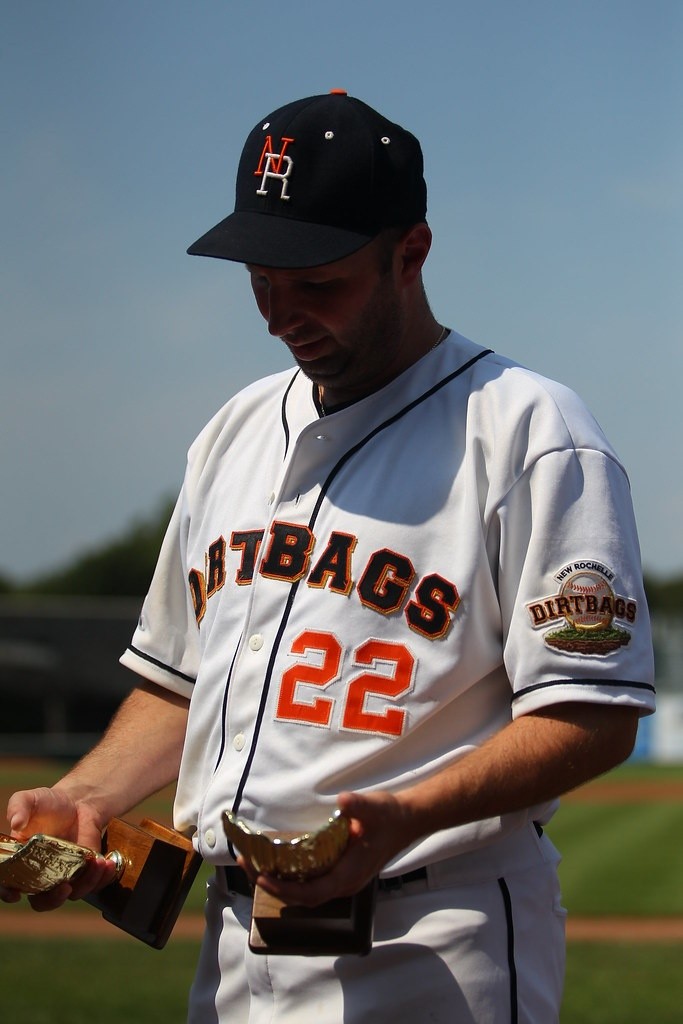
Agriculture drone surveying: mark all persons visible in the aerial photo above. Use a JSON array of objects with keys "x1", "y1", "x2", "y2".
[{"x1": 10, "y1": 86, "x2": 660, "y2": 1024}]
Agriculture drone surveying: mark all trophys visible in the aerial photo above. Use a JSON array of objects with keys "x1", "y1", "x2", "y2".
[
  {"x1": 216, "y1": 806, "x2": 379, "y2": 957},
  {"x1": 0, "y1": 815, "x2": 201, "y2": 951}
]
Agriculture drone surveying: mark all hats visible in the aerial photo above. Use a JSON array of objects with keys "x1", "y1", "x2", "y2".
[{"x1": 185, "y1": 89, "x2": 427, "y2": 265}]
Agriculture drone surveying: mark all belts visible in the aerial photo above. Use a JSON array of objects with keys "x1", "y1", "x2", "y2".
[{"x1": 224, "y1": 822, "x2": 544, "y2": 902}]
